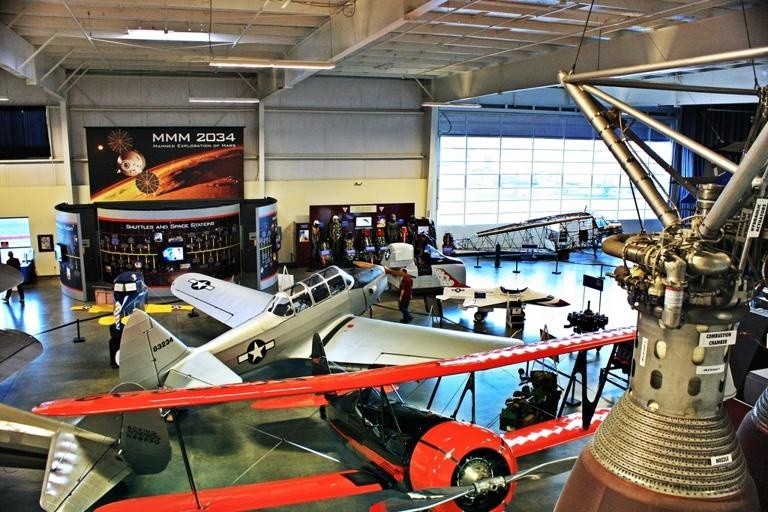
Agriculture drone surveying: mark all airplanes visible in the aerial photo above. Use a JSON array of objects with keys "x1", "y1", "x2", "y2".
[
  {"x1": 433, "y1": 267, "x2": 571, "y2": 322},
  {"x1": 113, "y1": 263, "x2": 526, "y2": 410},
  {"x1": 0, "y1": 328, "x2": 176, "y2": 512},
  {"x1": 30, "y1": 323, "x2": 647, "y2": 512}
]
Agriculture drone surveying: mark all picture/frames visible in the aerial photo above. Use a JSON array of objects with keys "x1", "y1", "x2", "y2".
[{"x1": 37, "y1": 234, "x2": 54, "y2": 252}]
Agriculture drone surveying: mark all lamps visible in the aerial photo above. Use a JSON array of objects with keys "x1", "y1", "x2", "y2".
[
  {"x1": 184, "y1": 61, "x2": 263, "y2": 105},
  {"x1": 208, "y1": 1, "x2": 337, "y2": 69}
]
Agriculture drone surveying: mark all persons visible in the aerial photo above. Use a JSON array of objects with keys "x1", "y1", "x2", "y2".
[
  {"x1": 397, "y1": 268, "x2": 415, "y2": 322},
  {"x1": 2, "y1": 251, "x2": 25, "y2": 302}
]
